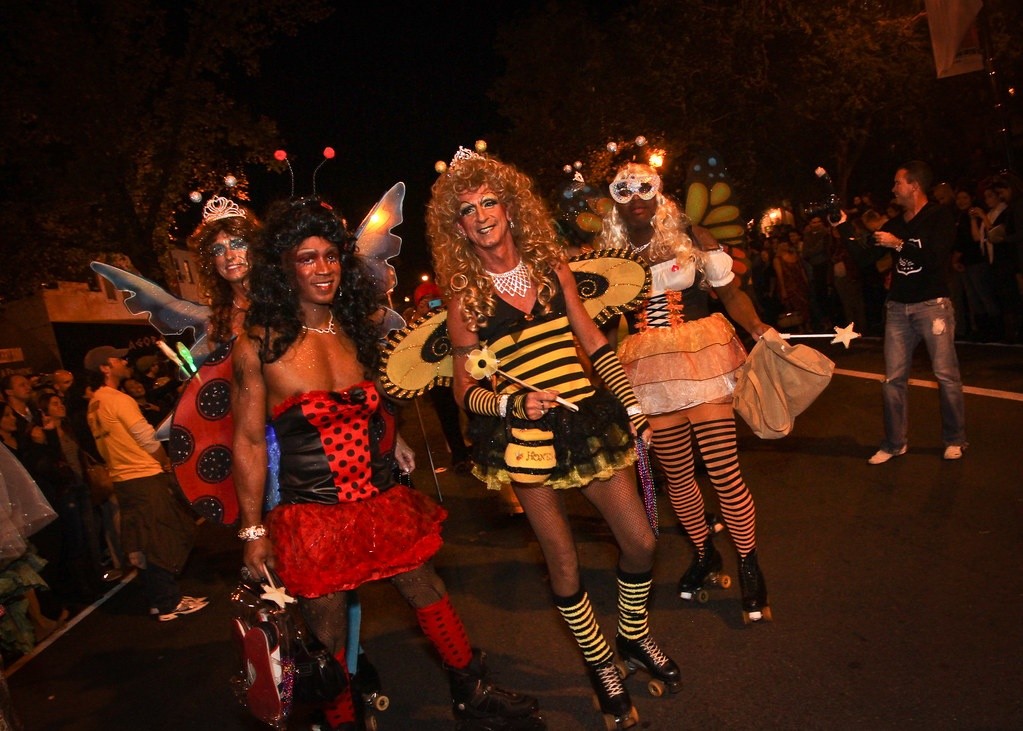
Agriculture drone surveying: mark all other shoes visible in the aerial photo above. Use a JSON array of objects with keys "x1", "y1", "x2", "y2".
[
  {"x1": 31, "y1": 616, "x2": 58, "y2": 642},
  {"x1": 944, "y1": 446, "x2": 962, "y2": 460},
  {"x1": 451, "y1": 450, "x2": 469, "y2": 473},
  {"x1": 676, "y1": 509, "x2": 725, "y2": 534},
  {"x1": 868, "y1": 444, "x2": 907, "y2": 465},
  {"x1": 101, "y1": 567, "x2": 123, "y2": 582}
]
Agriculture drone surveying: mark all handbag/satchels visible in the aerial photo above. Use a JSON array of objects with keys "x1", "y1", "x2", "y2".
[
  {"x1": 731, "y1": 326, "x2": 836, "y2": 440},
  {"x1": 266, "y1": 566, "x2": 347, "y2": 703},
  {"x1": 78, "y1": 450, "x2": 114, "y2": 508},
  {"x1": 229, "y1": 562, "x2": 294, "y2": 731},
  {"x1": 778, "y1": 302, "x2": 804, "y2": 327}
]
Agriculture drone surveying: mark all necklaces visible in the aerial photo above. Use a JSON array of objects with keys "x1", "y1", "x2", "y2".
[
  {"x1": 232, "y1": 299, "x2": 240, "y2": 308},
  {"x1": 484, "y1": 255, "x2": 533, "y2": 298},
  {"x1": 630, "y1": 240, "x2": 651, "y2": 253},
  {"x1": 301, "y1": 309, "x2": 337, "y2": 335}
]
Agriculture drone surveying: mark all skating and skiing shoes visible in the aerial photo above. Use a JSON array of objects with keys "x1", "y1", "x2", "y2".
[
  {"x1": 615, "y1": 629, "x2": 683, "y2": 697},
  {"x1": 310, "y1": 692, "x2": 378, "y2": 731},
  {"x1": 445, "y1": 647, "x2": 546, "y2": 731},
  {"x1": 736, "y1": 547, "x2": 772, "y2": 625},
  {"x1": 349, "y1": 653, "x2": 389, "y2": 711},
  {"x1": 588, "y1": 654, "x2": 639, "y2": 731},
  {"x1": 678, "y1": 535, "x2": 732, "y2": 604}
]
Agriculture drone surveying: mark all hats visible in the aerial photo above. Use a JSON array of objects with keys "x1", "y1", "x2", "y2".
[{"x1": 84, "y1": 346, "x2": 129, "y2": 373}]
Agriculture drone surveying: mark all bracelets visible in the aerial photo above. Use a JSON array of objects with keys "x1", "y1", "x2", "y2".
[
  {"x1": 895, "y1": 239, "x2": 904, "y2": 252},
  {"x1": 236, "y1": 524, "x2": 266, "y2": 543}
]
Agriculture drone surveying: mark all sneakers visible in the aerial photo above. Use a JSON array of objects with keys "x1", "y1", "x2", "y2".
[
  {"x1": 159, "y1": 596, "x2": 210, "y2": 622},
  {"x1": 150, "y1": 601, "x2": 160, "y2": 614}
]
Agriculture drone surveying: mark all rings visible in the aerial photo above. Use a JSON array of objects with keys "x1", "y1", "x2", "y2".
[
  {"x1": 541, "y1": 401, "x2": 544, "y2": 409},
  {"x1": 647, "y1": 441, "x2": 651, "y2": 444},
  {"x1": 240, "y1": 566, "x2": 251, "y2": 577},
  {"x1": 540, "y1": 410, "x2": 546, "y2": 415}
]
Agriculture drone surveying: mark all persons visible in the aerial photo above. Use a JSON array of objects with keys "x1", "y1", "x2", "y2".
[
  {"x1": 745, "y1": 169, "x2": 1023, "y2": 346},
  {"x1": 184, "y1": 135, "x2": 777, "y2": 731},
  {"x1": 0, "y1": 344, "x2": 209, "y2": 672},
  {"x1": 866, "y1": 162, "x2": 972, "y2": 465}
]
